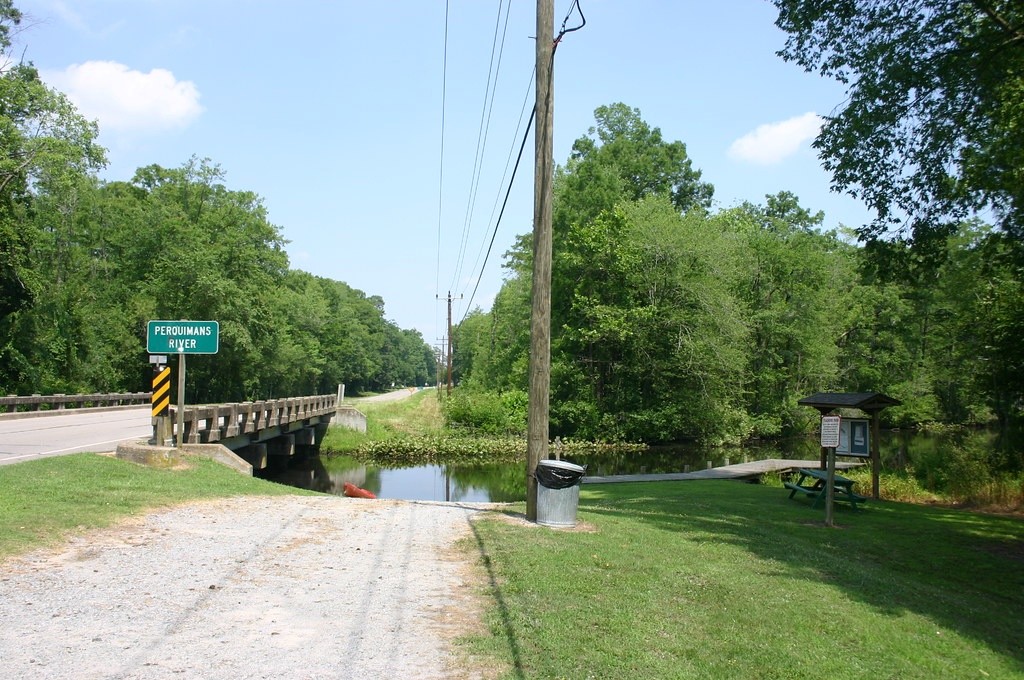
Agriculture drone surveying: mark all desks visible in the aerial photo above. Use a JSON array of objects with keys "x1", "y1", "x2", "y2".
[{"x1": 788, "y1": 469, "x2": 858, "y2": 511}]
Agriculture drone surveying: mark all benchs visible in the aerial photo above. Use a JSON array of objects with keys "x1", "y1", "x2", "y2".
[
  {"x1": 784, "y1": 481, "x2": 814, "y2": 495},
  {"x1": 835, "y1": 486, "x2": 866, "y2": 500}
]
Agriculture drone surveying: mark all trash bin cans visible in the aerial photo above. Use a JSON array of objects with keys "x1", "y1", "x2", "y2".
[{"x1": 534, "y1": 459, "x2": 586, "y2": 529}]
